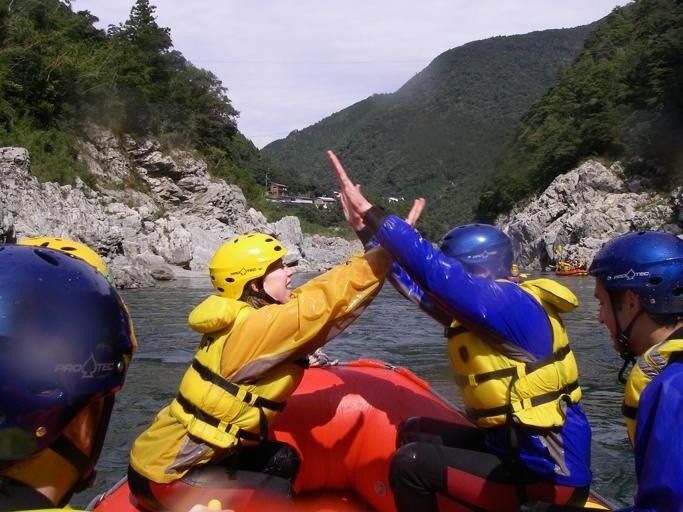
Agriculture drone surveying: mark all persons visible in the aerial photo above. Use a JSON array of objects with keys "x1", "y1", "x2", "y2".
[
  {"x1": 589, "y1": 228, "x2": 682, "y2": 511},
  {"x1": 11, "y1": 238, "x2": 116, "y2": 293},
  {"x1": 0, "y1": 244, "x2": 132, "y2": 512},
  {"x1": 326, "y1": 148, "x2": 590, "y2": 511},
  {"x1": 129, "y1": 198, "x2": 425, "y2": 511},
  {"x1": 557, "y1": 258, "x2": 586, "y2": 272},
  {"x1": 248, "y1": 337, "x2": 285, "y2": 340}
]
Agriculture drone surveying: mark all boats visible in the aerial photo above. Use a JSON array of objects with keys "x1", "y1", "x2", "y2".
[
  {"x1": 84, "y1": 357, "x2": 621, "y2": 512},
  {"x1": 511, "y1": 276, "x2": 520, "y2": 283},
  {"x1": 554, "y1": 266, "x2": 589, "y2": 277}
]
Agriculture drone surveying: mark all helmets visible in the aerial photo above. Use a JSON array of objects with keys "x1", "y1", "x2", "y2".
[
  {"x1": 0, "y1": 236, "x2": 135, "y2": 462},
  {"x1": 209, "y1": 232, "x2": 286, "y2": 300},
  {"x1": 440, "y1": 223, "x2": 516, "y2": 283},
  {"x1": 587, "y1": 231, "x2": 682, "y2": 314}
]
